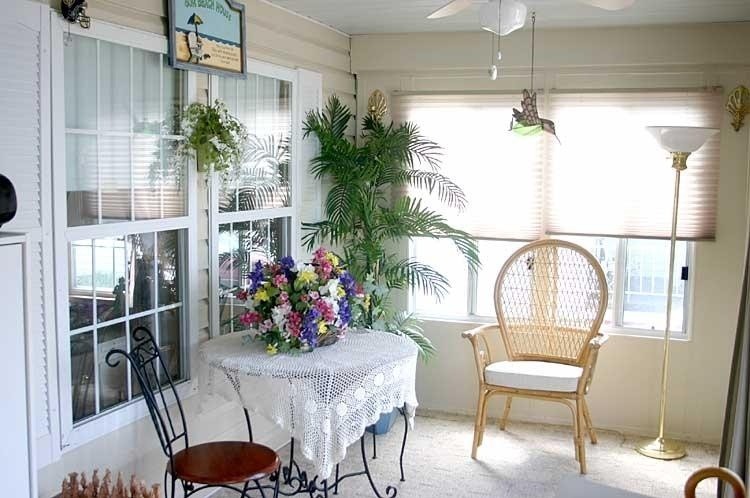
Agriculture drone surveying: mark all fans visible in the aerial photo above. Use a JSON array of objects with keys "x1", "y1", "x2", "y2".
[{"x1": 426, "y1": 1, "x2": 637, "y2": 20}]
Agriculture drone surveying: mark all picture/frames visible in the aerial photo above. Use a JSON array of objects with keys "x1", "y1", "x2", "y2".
[{"x1": 167, "y1": 0, "x2": 248, "y2": 79}]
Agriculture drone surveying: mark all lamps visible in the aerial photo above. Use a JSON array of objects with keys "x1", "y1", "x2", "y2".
[
  {"x1": 634, "y1": 126, "x2": 721, "y2": 461},
  {"x1": 479, "y1": 1, "x2": 527, "y2": 37}
]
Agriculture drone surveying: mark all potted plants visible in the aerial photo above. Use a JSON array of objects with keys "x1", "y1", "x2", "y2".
[
  {"x1": 299, "y1": 92, "x2": 482, "y2": 435},
  {"x1": 173, "y1": 97, "x2": 248, "y2": 195}
]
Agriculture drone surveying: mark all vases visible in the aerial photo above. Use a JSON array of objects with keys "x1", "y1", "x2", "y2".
[{"x1": 314, "y1": 328, "x2": 339, "y2": 347}]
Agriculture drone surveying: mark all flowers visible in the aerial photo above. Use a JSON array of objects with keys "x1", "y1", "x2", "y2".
[{"x1": 233, "y1": 248, "x2": 373, "y2": 357}]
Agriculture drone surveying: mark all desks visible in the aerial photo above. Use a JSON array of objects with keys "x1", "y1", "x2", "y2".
[{"x1": 197, "y1": 328, "x2": 420, "y2": 498}]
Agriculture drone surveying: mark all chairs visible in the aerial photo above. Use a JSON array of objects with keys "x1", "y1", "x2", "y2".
[
  {"x1": 104, "y1": 326, "x2": 281, "y2": 498},
  {"x1": 372, "y1": 401, "x2": 408, "y2": 481},
  {"x1": 460, "y1": 238, "x2": 610, "y2": 474}
]
